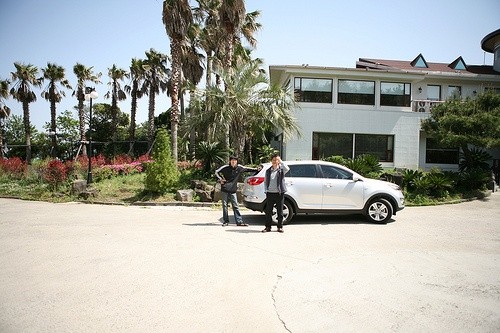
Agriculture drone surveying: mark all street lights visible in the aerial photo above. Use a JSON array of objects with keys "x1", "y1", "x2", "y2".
[{"x1": 84, "y1": 91, "x2": 99, "y2": 184}]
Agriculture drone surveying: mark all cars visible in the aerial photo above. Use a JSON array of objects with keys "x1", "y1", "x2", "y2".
[{"x1": 243, "y1": 160, "x2": 405, "y2": 225}]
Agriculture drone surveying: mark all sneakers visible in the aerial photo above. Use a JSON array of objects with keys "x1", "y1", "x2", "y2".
[
  {"x1": 262, "y1": 228, "x2": 271, "y2": 232},
  {"x1": 277, "y1": 228, "x2": 284, "y2": 232}
]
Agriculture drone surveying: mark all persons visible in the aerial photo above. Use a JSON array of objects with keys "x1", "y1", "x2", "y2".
[
  {"x1": 261, "y1": 154, "x2": 290, "y2": 233},
  {"x1": 214, "y1": 157, "x2": 262, "y2": 227}
]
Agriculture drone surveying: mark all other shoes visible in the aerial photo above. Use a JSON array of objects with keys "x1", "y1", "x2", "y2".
[
  {"x1": 237, "y1": 223, "x2": 249, "y2": 226},
  {"x1": 222, "y1": 222, "x2": 228, "y2": 226}
]
